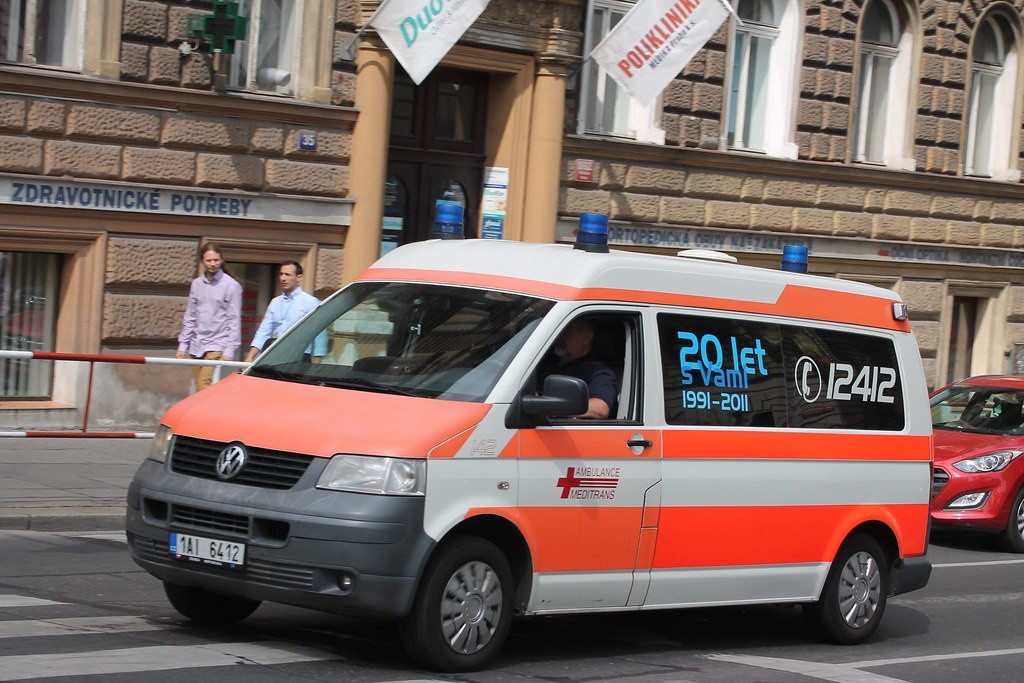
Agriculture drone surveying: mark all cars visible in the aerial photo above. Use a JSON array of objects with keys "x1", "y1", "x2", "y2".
[{"x1": 927, "y1": 371, "x2": 1024, "y2": 553}]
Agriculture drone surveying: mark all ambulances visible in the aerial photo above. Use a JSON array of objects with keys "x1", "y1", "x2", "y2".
[{"x1": 123, "y1": 189, "x2": 937, "y2": 671}]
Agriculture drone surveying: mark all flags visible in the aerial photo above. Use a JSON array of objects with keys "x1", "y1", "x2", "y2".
[
  {"x1": 590, "y1": 0, "x2": 732, "y2": 109},
  {"x1": 371, "y1": 0, "x2": 490, "y2": 86}
]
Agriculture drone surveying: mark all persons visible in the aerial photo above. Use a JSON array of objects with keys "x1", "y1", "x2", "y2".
[
  {"x1": 540, "y1": 316, "x2": 620, "y2": 421},
  {"x1": 245, "y1": 260, "x2": 329, "y2": 364},
  {"x1": 177, "y1": 241, "x2": 244, "y2": 394}
]
